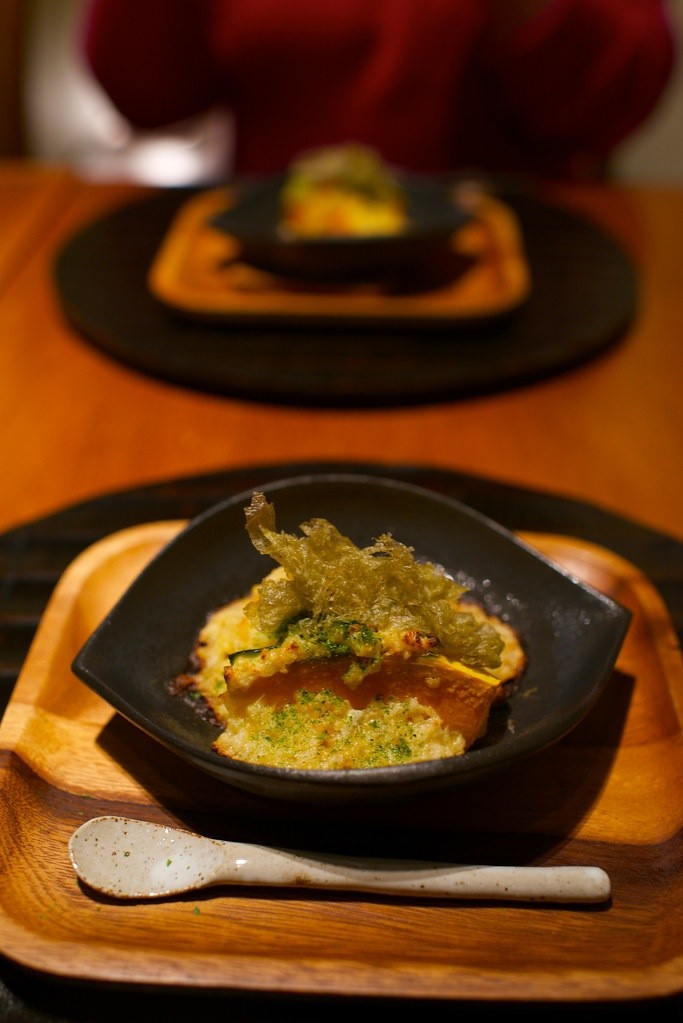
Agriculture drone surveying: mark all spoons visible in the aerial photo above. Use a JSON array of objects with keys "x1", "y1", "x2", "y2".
[{"x1": 61, "y1": 808, "x2": 610, "y2": 916}]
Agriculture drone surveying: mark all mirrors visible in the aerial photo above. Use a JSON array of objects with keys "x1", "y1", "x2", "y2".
[{"x1": 0, "y1": 2, "x2": 683, "y2": 555}]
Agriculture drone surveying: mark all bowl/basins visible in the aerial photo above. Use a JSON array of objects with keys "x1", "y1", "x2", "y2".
[
  {"x1": 209, "y1": 175, "x2": 475, "y2": 275},
  {"x1": 72, "y1": 455, "x2": 632, "y2": 802}
]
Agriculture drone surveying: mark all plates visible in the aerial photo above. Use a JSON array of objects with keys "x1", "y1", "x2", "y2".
[
  {"x1": 0, "y1": 450, "x2": 682, "y2": 1022},
  {"x1": 149, "y1": 190, "x2": 525, "y2": 320},
  {"x1": 54, "y1": 166, "x2": 642, "y2": 407},
  {"x1": 3, "y1": 513, "x2": 679, "y2": 1003}
]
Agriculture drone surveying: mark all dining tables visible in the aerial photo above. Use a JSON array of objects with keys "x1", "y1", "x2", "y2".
[{"x1": 0, "y1": 156, "x2": 683, "y2": 1023}]
